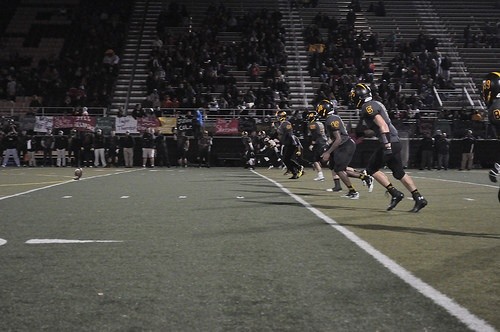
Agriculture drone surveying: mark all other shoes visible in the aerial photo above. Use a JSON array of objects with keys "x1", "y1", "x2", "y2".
[
  {"x1": 488, "y1": 168, "x2": 497, "y2": 183},
  {"x1": 408, "y1": 191, "x2": 428, "y2": 212},
  {"x1": 267, "y1": 165, "x2": 274, "y2": 170},
  {"x1": 366, "y1": 176, "x2": 374, "y2": 192},
  {"x1": 314, "y1": 177, "x2": 324, "y2": 182},
  {"x1": 359, "y1": 169, "x2": 367, "y2": 186},
  {"x1": 282, "y1": 165, "x2": 286, "y2": 171},
  {"x1": 297, "y1": 165, "x2": 304, "y2": 177},
  {"x1": 385, "y1": 187, "x2": 404, "y2": 211},
  {"x1": 341, "y1": 191, "x2": 359, "y2": 199},
  {"x1": 288, "y1": 175, "x2": 298, "y2": 179},
  {"x1": 384, "y1": 143, "x2": 392, "y2": 154},
  {"x1": 326, "y1": 186, "x2": 342, "y2": 191}
]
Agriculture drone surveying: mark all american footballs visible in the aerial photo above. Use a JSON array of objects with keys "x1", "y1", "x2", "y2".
[{"x1": 74, "y1": 167, "x2": 83, "y2": 178}]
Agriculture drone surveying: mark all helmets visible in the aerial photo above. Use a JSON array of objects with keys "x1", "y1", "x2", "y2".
[
  {"x1": 276, "y1": 110, "x2": 287, "y2": 122},
  {"x1": 302, "y1": 109, "x2": 317, "y2": 124},
  {"x1": 242, "y1": 131, "x2": 248, "y2": 137},
  {"x1": 350, "y1": 83, "x2": 372, "y2": 109},
  {"x1": 258, "y1": 131, "x2": 265, "y2": 136},
  {"x1": 480, "y1": 72, "x2": 500, "y2": 109},
  {"x1": 316, "y1": 100, "x2": 334, "y2": 118}
]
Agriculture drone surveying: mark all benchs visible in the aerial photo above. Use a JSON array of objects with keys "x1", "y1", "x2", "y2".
[{"x1": 0, "y1": 0, "x2": 500, "y2": 113}]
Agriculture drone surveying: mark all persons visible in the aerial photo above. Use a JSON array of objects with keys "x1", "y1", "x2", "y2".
[
  {"x1": 459, "y1": 129, "x2": 477, "y2": 170},
  {"x1": 197, "y1": 130, "x2": 213, "y2": 168},
  {"x1": 27, "y1": 139, "x2": 39, "y2": 166},
  {"x1": 419, "y1": 132, "x2": 450, "y2": 171},
  {"x1": 121, "y1": 130, "x2": 136, "y2": 167},
  {"x1": 258, "y1": 100, "x2": 374, "y2": 199},
  {"x1": 69, "y1": 128, "x2": 93, "y2": 167},
  {"x1": 93, "y1": 128, "x2": 106, "y2": 167},
  {"x1": 241, "y1": 130, "x2": 255, "y2": 170},
  {"x1": 0, "y1": 0, "x2": 500, "y2": 138},
  {"x1": 481, "y1": 72, "x2": 500, "y2": 200},
  {"x1": 177, "y1": 131, "x2": 189, "y2": 167},
  {"x1": 2, "y1": 131, "x2": 21, "y2": 168},
  {"x1": 41, "y1": 128, "x2": 55, "y2": 167},
  {"x1": 54, "y1": 131, "x2": 68, "y2": 167},
  {"x1": 350, "y1": 83, "x2": 427, "y2": 213},
  {"x1": 107, "y1": 130, "x2": 119, "y2": 168},
  {"x1": 141, "y1": 128, "x2": 155, "y2": 168}
]
